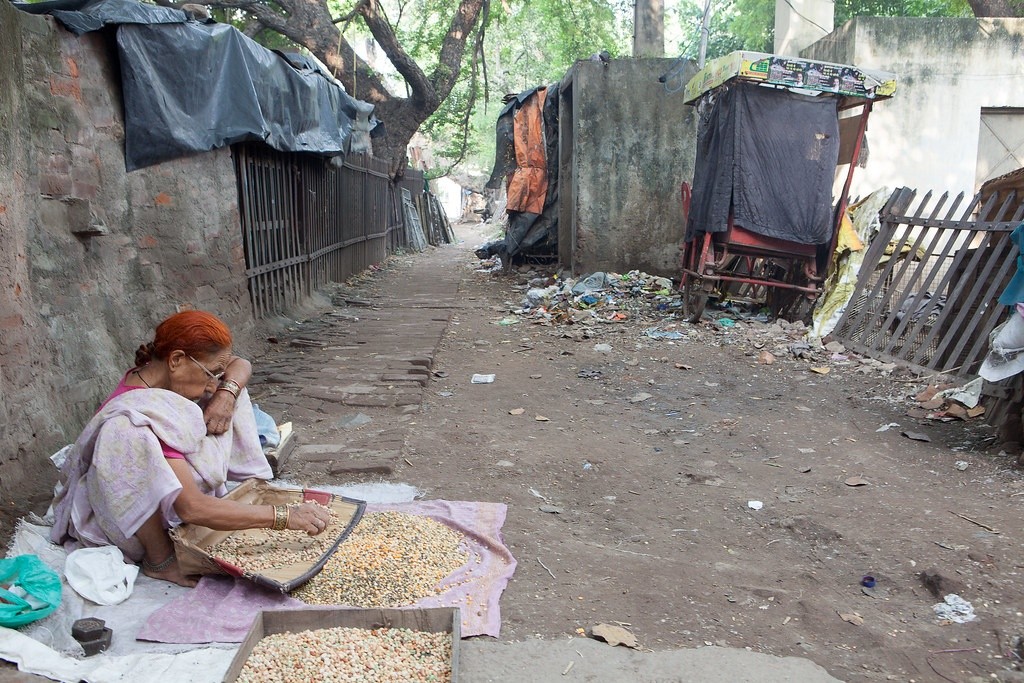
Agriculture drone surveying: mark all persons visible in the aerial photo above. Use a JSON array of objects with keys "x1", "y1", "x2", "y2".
[{"x1": 92, "y1": 310, "x2": 329, "y2": 588}]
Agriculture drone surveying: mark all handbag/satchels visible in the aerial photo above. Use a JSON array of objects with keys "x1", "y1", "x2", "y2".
[
  {"x1": 0, "y1": 554, "x2": 62, "y2": 629},
  {"x1": 65, "y1": 546, "x2": 140, "y2": 606}
]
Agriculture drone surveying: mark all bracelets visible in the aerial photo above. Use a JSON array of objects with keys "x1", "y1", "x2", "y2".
[
  {"x1": 218, "y1": 379, "x2": 240, "y2": 398},
  {"x1": 272, "y1": 505, "x2": 289, "y2": 531}
]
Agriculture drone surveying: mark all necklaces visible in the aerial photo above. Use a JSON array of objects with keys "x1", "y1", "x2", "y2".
[{"x1": 136, "y1": 371, "x2": 150, "y2": 388}]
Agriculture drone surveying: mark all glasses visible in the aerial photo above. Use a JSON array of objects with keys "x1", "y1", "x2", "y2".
[{"x1": 185, "y1": 353, "x2": 225, "y2": 383}]
